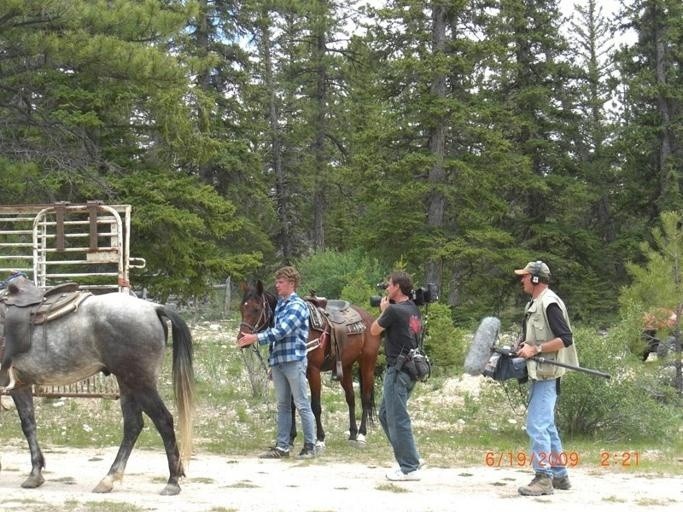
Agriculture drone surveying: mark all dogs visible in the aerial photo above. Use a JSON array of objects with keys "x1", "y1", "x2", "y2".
[
  {"x1": 237, "y1": 280, "x2": 381, "y2": 449},
  {"x1": 0, "y1": 275, "x2": 194, "y2": 497}
]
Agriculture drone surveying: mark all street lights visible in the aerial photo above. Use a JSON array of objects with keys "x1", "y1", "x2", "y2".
[
  {"x1": 406, "y1": 349, "x2": 431, "y2": 382},
  {"x1": 492, "y1": 352, "x2": 528, "y2": 381}
]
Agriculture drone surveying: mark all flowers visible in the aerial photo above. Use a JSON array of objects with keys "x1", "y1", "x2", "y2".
[{"x1": 463, "y1": 316, "x2": 501, "y2": 375}]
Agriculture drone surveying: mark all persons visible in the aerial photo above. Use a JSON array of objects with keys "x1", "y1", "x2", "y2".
[
  {"x1": 369, "y1": 270, "x2": 426, "y2": 481},
  {"x1": 514, "y1": 260, "x2": 580, "y2": 496},
  {"x1": 239, "y1": 267, "x2": 320, "y2": 460}
]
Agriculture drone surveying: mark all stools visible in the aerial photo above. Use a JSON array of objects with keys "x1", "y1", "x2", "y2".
[{"x1": 556, "y1": 377, "x2": 560, "y2": 394}]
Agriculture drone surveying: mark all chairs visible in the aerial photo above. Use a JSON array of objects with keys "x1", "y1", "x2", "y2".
[
  {"x1": 259, "y1": 447, "x2": 290, "y2": 460},
  {"x1": 553, "y1": 474, "x2": 570, "y2": 491},
  {"x1": 386, "y1": 471, "x2": 422, "y2": 482},
  {"x1": 418, "y1": 458, "x2": 428, "y2": 472},
  {"x1": 297, "y1": 444, "x2": 316, "y2": 459}
]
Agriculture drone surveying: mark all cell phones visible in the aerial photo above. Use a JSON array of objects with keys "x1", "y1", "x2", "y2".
[{"x1": 370, "y1": 282, "x2": 438, "y2": 307}]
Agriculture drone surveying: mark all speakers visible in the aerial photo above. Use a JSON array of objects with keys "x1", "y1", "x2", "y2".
[{"x1": 518, "y1": 472, "x2": 554, "y2": 497}]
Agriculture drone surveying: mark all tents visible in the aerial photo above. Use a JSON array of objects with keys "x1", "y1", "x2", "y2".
[{"x1": 530, "y1": 260, "x2": 542, "y2": 284}]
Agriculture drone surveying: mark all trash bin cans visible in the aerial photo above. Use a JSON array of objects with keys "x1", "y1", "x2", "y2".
[{"x1": 536, "y1": 344, "x2": 543, "y2": 354}]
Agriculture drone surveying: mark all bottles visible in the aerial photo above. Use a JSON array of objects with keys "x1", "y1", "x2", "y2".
[{"x1": 513, "y1": 262, "x2": 551, "y2": 283}]
